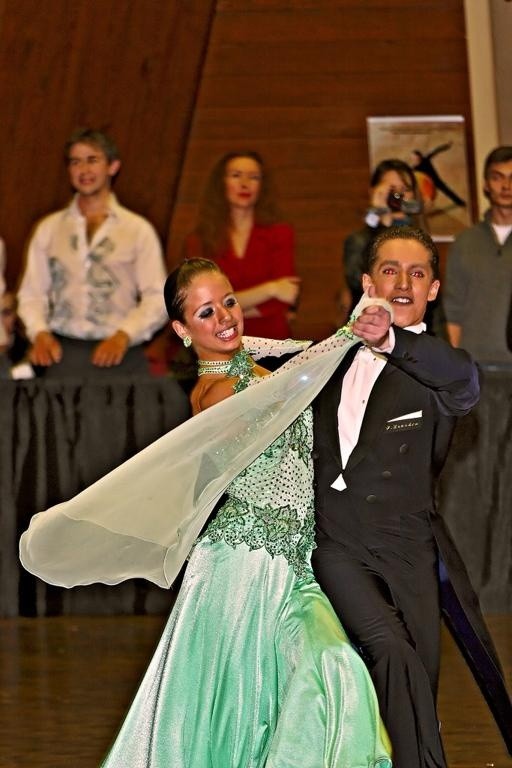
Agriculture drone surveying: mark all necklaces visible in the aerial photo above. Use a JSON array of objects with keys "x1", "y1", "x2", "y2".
[{"x1": 196, "y1": 350, "x2": 255, "y2": 376}]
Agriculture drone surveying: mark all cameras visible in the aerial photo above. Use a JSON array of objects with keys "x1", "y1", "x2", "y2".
[{"x1": 384, "y1": 188, "x2": 423, "y2": 221}]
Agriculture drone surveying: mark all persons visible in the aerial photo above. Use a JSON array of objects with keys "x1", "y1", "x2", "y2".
[
  {"x1": 183, "y1": 150, "x2": 302, "y2": 338},
  {"x1": 257, "y1": 224, "x2": 481, "y2": 767},
  {"x1": 0, "y1": 234, "x2": 29, "y2": 368},
  {"x1": 442, "y1": 145, "x2": 511, "y2": 368},
  {"x1": 16, "y1": 125, "x2": 168, "y2": 383},
  {"x1": 104, "y1": 256, "x2": 394, "y2": 767},
  {"x1": 342, "y1": 159, "x2": 448, "y2": 341}
]
